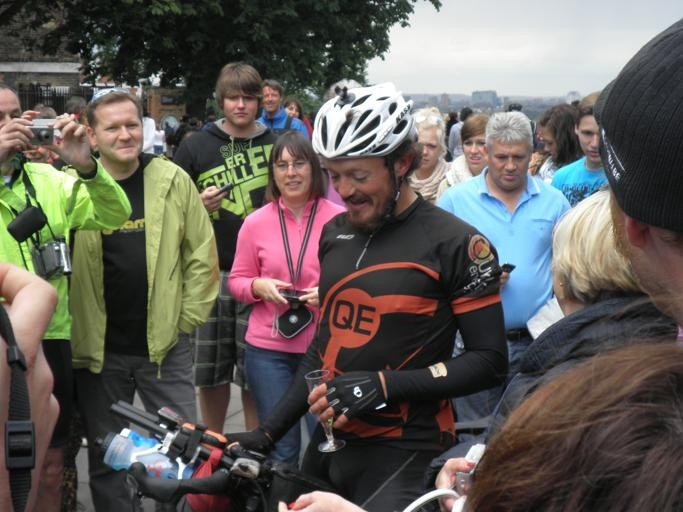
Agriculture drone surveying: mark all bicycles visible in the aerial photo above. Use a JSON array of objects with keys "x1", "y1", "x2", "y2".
[{"x1": 96, "y1": 400, "x2": 334, "y2": 511}]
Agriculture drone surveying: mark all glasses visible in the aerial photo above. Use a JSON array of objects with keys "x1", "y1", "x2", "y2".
[{"x1": 275, "y1": 160, "x2": 307, "y2": 173}]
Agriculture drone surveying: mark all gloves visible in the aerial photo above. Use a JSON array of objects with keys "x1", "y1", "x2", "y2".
[{"x1": 318, "y1": 366, "x2": 393, "y2": 428}]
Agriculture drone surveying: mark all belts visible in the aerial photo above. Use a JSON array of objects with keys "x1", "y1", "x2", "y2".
[{"x1": 504, "y1": 331, "x2": 530, "y2": 343}]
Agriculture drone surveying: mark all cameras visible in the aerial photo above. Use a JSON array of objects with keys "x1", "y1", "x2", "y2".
[
  {"x1": 455, "y1": 443, "x2": 486, "y2": 497},
  {"x1": 279, "y1": 288, "x2": 309, "y2": 310},
  {"x1": 25, "y1": 126, "x2": 53, "y2": 146}
]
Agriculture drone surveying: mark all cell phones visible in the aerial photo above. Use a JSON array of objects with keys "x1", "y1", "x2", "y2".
[
  {"x1": 501, "y1": 263, "x2": 516, "y2": 272},
  {"x1": 219, "y1": 183, "x2": 234, "y2": 193}
]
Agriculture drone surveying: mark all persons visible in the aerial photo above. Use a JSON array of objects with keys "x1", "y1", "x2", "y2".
[
  {"x1": 427, "y1": 191, "x2": 682, "y2": 511},
  {"x1": 445, "y1": 112, "x2": 459, "y2": 143},
  {"x1": 439, "y1": 113, "x2": 576, "y2": 446},
  {"x1": 282, "y1": 98, "x2": 313, "y2": 140},
  {"x1": 463, "y1": 342, "x2": 681, "y2": 511},
  {"x1": 1, "y1": 81, "x2": 131, "y2": 512},
  {"x1": 171, "y1": 65, "x2": 279, "y2": 435},
  {"x1": 454, "y1": 107, "x2": 475, "y2": 157},
  {"x1": 15, "y1": 107, "x2": 66, "y2": 170},
  {"x1": 435, "y1": 115, "x2": 490, "y2": 201},
  {"x1": 405, "y1": 106, "x2": 452, "y2": 200},
  {"x1": 73, "y1": 92, "x2": 218, "y2": 512},
  {"x1": 258, "y1": 80, "x2": 308, "y2": 142},
  {"x1": 1, "y1": 259, "x2": 61, "y2": 512},
  {"x1": 552, "y1": 92, "x2": 612, "y2": 205},
  {"x1": 449, "y1": 13, "x2": 683, "y2": 338},
  {"x1": 229, "y1": 133, "x2": 350, "y2": 505},
  {"x1": 228, "y1": 83, "x2": 510, "y2": 512},
  {"x1": 533, "y1": 104, "x2": 582, "y2": 183}
]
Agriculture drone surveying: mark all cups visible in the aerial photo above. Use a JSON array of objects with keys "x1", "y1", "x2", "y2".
[{"x1": 304, "y1": 370, "x2": 346, "y2": 453}]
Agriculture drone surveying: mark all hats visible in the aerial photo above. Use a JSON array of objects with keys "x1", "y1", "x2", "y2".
[
  {"x1": 595, "y1": 19, "x2": 683, "y2": 231},
  {"x1": 311, "y1": 82, "x2": 417, "y2": 162}
]
